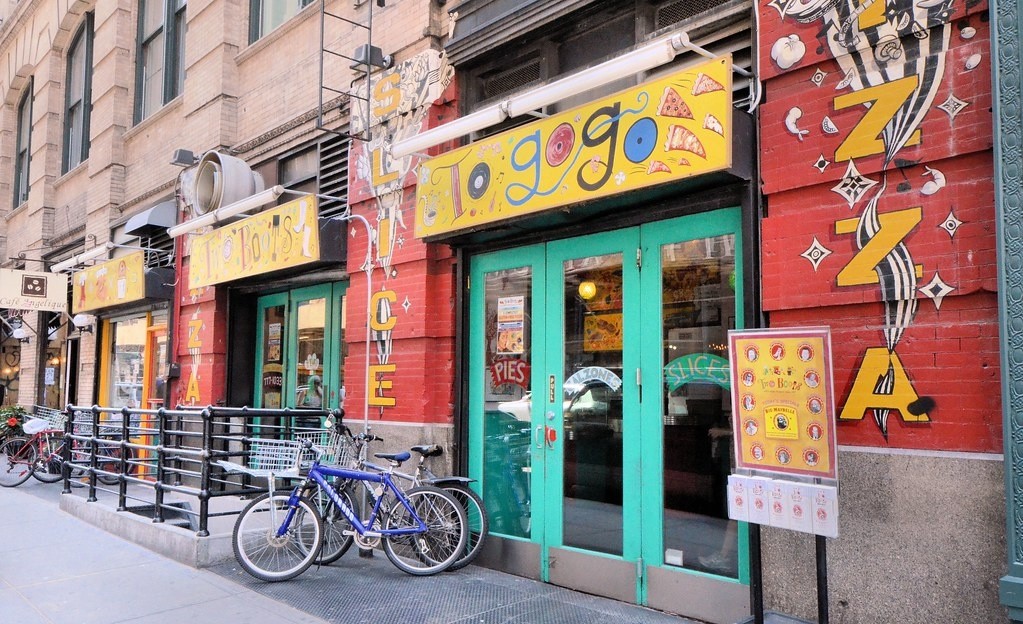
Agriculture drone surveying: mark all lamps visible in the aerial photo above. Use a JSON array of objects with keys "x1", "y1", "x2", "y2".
[
  {"x1": 349, "y1": 45, "x2": 394, "y2": 74},
  {"x1": 13, "y1": 327, "x2": 30, "y2": 344},
  {"x1": 166, "y1": 185, "x2": 347, "y2": 238},
  {"x1": 73, "y1": 312, "x2": 94, "y2": 335},
  {"x1": 388, "y1": 32, "x2": 754, "y2": 160},
  {"x1": 169, "y1": 149, "x2": 199, "y2": 167},
  {"x1": 50, "y1": 242, "x2": 173, "y2": 273}
]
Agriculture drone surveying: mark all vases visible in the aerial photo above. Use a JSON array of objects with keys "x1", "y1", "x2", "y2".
[{"x1": 4, "y1": 440, "x2": 29, "y2": 459}]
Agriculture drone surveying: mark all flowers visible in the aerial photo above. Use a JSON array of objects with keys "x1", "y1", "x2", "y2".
[{"x1": 0, "y1": 406, "x2": 31, "y2": 438}]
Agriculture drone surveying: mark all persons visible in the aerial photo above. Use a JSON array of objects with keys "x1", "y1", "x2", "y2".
[
  {"x1": 135, "y1": 362, "x2": 165, "y2": 445},
  {"x1": 698, "y1": 412, "x2": 738, "y2": 574}
]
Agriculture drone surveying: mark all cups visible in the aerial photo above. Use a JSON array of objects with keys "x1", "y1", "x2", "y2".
[{"x1": 116, "y1": 277, "x2": 127, "y2": 299}]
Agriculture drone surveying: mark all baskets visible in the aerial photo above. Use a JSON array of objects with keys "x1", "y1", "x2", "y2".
[
  {"x1": 295, "y1": 430, "x2": 352, "y2": 469},
  {"x1": 248, "y1": 437, "x2": 304, "y2": 477},
  {"x1": 35, "y1": 405, "x2": 66, "y2": 431},
  {"x1": 73, "y1": 412, "x2": 94, "y2": 434}
]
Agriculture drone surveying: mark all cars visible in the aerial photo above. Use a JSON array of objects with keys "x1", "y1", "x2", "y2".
[{"x1": 496, "y1": 383, "x2": 611, "y2": 424}]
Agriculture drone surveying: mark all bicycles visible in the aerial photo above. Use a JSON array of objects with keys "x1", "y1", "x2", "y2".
[
  {"x1": 295, "y1": 421, "x2": 489, "y2": 573},
  {"x1": 1, "y1": 404, "x2": 138, "y2": 488},
  {"x1": 232, "y1": 429, "x2": 469, "y2": 583}
]
